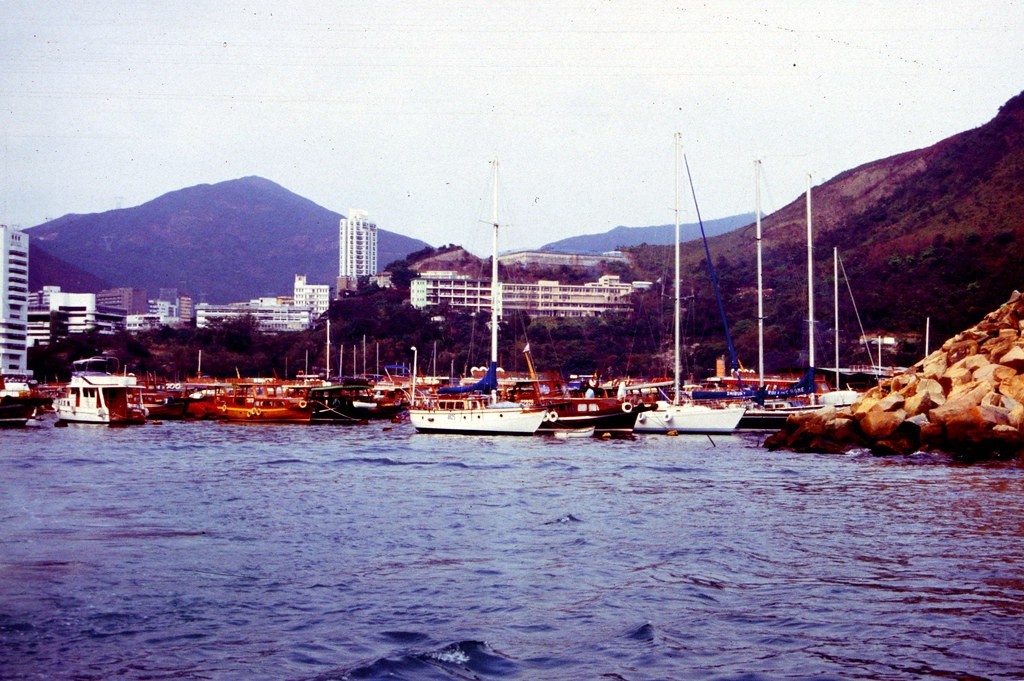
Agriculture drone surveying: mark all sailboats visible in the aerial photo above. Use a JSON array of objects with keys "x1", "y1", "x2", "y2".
[
  {"x1": 620, "y1": 106, "x2": 747, "y2": 435},
  {"x1": 722, "y1": 155, "x2": 881, "y2": 435},
  {"x1": 408, "y1": 156, "x2": 574, "y2": 435}
]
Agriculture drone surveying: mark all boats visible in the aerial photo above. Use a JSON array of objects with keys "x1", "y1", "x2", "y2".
[
  {"x1": 0, "y1": 379, "x2": 54, "y2": 429},
  {"x1": 127, "y1": 314, "x2": 829, "y2": 426},
  {"x1": 52, "y1": 357, "x2": 149, "y2": 424}
]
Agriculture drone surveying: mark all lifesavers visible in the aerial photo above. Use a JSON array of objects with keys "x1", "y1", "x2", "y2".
[
  {"x1": 638, "y1": 412, "x2": 646, "y2": 422},
  {"x1": 663, "y1": 413, "x2": 673, "y2": 422},
  {"x1": 55, "y1": 405, "x2": 60, "y2": 412},
  {"x1": 97, "y1": 408, "x2": 105, "y2": 417},
  {"x1": 622, "y1": 402, "x2": 633, "y2": 413},
  {"x1": 165, "y1": 383, "x2": 170, "y2": 389},
  {"x1": 542, "y1": 412, "x2": 549, "y2": 422},
  {"x1": 176, "y1": 383, "x2": 181, "y2": 389},
  {"x1": 71, "y1": 405, "x2": 76, "y2": 413},
  {"x1": 222, "y1": 405, "x2": 227, "y2": 411},
  {"x1": 246, "y1": 411, "x2": 251, "y2": 417},
  {"x1": 251, "y1": 408, "x2": 256, "y2": 414},
  {"x1": 256, "y1": 408, "x2": 261, "y2": 415},
  {"x1": 549, "y1": 411, "x2": 558, "y2": 422},
  {"x1": 298, "y1": 400, "x2": 307, "y2": 408},
  {"x1": 170, "y1": 383, "x2": 175, "y2": 390}
]
